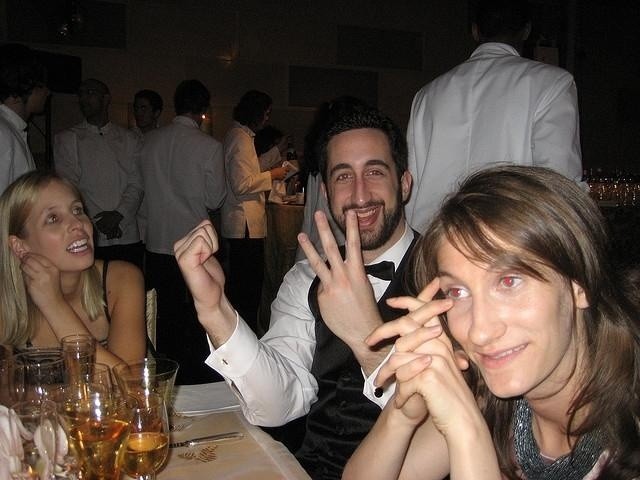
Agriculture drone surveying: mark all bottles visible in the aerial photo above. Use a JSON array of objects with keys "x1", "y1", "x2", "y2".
[
  {"x1": 295, "y1": 180, "x2": 306, "y2": 206},
  {"x1": 286, "y1": 135, "x2": 298, "y2": 161},
  {"x1": 581, "y1": 168, "x2": 640, "y2": 206}
]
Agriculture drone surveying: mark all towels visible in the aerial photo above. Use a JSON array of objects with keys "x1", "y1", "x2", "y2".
[
  {"x1": 172, "y1": 381, "x2": 240, "y2": 417},
  {"x1": 269, "y1": 161, "x2": 298, "y2": 205}
]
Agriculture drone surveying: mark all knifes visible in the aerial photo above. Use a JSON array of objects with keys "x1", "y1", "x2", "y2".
[{"x1": 169, "y1": 431, "x2": 245, "y2": 448}]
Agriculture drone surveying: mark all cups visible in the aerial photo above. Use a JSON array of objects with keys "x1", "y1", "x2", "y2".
[{"x1": 0, "y1": 332, "x2": 180, "y2": 480}]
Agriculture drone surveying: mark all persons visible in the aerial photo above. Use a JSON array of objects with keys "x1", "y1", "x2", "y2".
[
  {"x1": 2, "y1": 43, "x2": 51, "y2": 193},
  {"x1": 339, "y1": 164, "x2": 638, "y2": 478},
  {"x1": 172, "y1": 105, "x2": 428, "y2": 478},
  {"x1": 128, "y1": 88, "x2": 164, "y2": 145},
  {"x1": 217, "y1": 88, "x2": 289, "y2": 340},
  {"x1": 400, "y1": 4, "x2": 591, "y2": 238},
  {"x1": 137, "y1": 78, "x2": 228, "y2": 387},
  {"x1": 54, "y1": 77, "x2": 144, "y2": 272},
  {"x1": 2, "y1": 169, "x2": 146, "y2": 384}
]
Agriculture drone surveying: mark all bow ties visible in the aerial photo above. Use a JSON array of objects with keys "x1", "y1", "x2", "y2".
[{"x1": 364, "y1": 261, "x2": 394, "y2": 280}]
